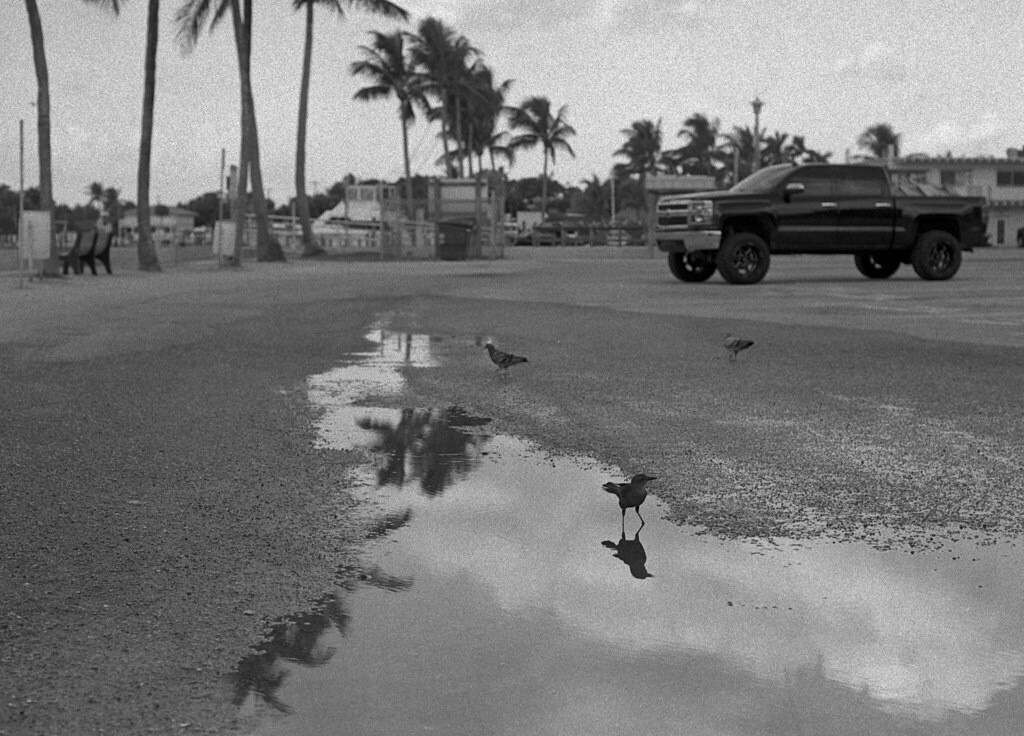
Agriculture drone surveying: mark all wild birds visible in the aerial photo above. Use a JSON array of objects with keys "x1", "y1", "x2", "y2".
[
  {"x1": 724, "y1": 333, "x2": 755, "y2": 360},
  {"x1": 602, "y1": 474, "x2": 658, "y2": 525},
  {"x1": 483, "y1": 343, "x2": 529, "y2": 372},
  {"x1": 601, "y1": 525, "x2": 654, "y2": 580}
]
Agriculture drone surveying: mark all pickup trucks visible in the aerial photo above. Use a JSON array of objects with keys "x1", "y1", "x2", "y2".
[{"x1": 654, "y1": 163, "x2": 989, "y2": 284}]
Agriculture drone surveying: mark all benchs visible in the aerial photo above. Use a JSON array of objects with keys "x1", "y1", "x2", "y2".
[{"x1": 58, "y1": 231, "x2": 113, "y2": 276}]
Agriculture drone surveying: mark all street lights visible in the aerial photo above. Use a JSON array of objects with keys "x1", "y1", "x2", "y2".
[{"x1": 752, "y1": 97, "x2": 763, "y2": 174}]
